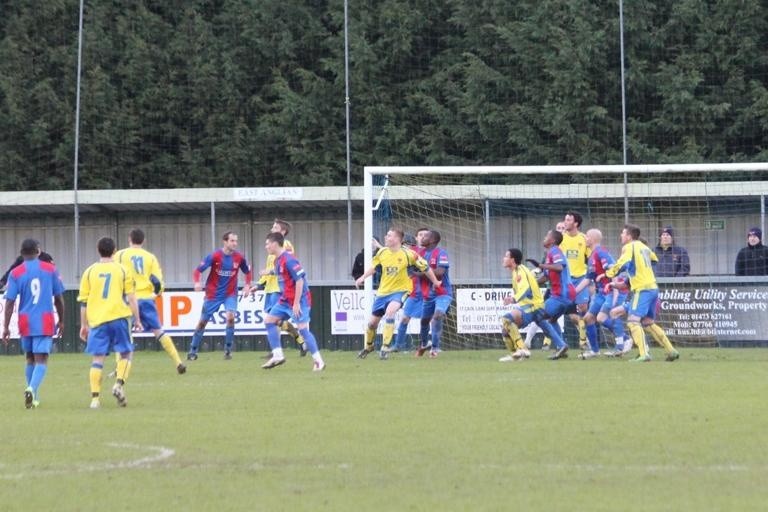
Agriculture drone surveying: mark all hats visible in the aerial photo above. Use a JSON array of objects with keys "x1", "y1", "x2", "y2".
[
  {"x1": 748, "y1": 228, "x2": 762, "y2": 240},
  {"x1": 659, "y1": 225, "x2": 673, "y2": 237}
]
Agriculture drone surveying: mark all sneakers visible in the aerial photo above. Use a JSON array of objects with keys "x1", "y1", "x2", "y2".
[
  {"x1": 500, "y1": 340, "x2": 678, "y2": 361},
  {"x1": 25, "y1": 387, "x2": 39, "y2": 409},
  {"x1": 89, "y1": 368, "x2": 126, "y2": 408},
  {"x1": 178, "y1": 354, "x2": 198, "y2": 373},
  {"x1": 261, "y1": 352, "x2": 284, "y2": 368},
  {"x1": 311, "y1": 361, "x2": 325, "y2": 373},
  {"x1": 300, "y1": 342, "x2": 308, "y2": 356},
  {"x1": 358, "y1": 341, "x2": 437, "y2": 359},
  {"x1": 224, "y1": 353, "x2": 232, "y2": 359}
]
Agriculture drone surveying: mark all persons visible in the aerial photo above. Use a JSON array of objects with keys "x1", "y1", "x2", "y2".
[
  {"x1": 187, "y1": 231, "x2": 252, "y2": 362},
  {"x1": 257, "y1": 231, "x2": 327, "y2": 372},
  {"x1": 732, "y1": 224, "x2": 768, "y2": 278},
  {"x1": 351, "y1": 228, "x2": 452, "y2": 359},
  {"x1": 75, "y1": 236, "x2": 144, "y2": 408},
  {"x1": 250, "y1": 218, "x2": 310, "y2": 361},
  {"x1": 3, "y1": 237, "x2": 66, "y2": 409},
  {"x1": 1, "y1": 239, "x2": 55, "y2": 293},
  {"x1": 500, "y1": 209, "x2": 680, "y2": 362},
  {"x1": 651, "y1": 222, "x2": 690, "y2": 277},
  {"x1": 108, "y1": 225, "x2": 187, "y2": 378}
]
